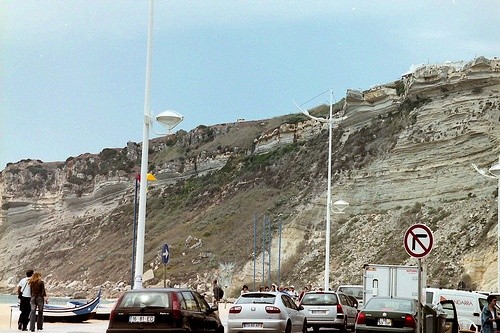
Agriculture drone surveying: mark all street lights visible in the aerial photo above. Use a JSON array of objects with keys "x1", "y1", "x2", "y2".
[
  {"x1": 133, "y1": 110, "x2": 184, "y2": 289},
  {"x1": 324, "y1": 200, "x2": 350, "y2": 291}
]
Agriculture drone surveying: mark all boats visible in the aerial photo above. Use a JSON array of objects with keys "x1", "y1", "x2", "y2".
[{"x1": 17, "y1": 289, "x2": 102, "y2": 320}]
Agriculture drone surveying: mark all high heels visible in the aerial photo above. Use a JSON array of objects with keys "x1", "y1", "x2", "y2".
[
  {"x1": 37, "y1": 328, "x2": 43, "y2": 330},
  {"x1": 29, "y1": 329, "x2": 35, "y2": 332}
]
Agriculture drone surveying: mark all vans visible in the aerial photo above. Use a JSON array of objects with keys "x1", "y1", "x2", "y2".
[{"x1": 424, "y1": 287, "x2": 500, "y2": 330}]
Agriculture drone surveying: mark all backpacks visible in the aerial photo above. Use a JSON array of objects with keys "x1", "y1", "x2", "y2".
[{"x1": 218, "y1": 288, "x2": 224, "y2": 299}]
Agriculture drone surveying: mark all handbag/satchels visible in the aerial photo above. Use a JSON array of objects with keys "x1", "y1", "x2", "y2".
[{"x1": 18, "y1": 292, "x2": 23, "y2": 299}]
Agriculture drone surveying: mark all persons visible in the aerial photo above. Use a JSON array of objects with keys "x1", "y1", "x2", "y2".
[
  {"x1": 481, "y1": 295, "x2": 500, "y2": 333},
  {"x1": 17, "y1": 270, "x2": 34, "y2": 331},
  {"x1": 28, "y1": 272, "x2": 49, "y2": 332},
  {"x1": 212, "y1": 279, "x2": 221, "y2": 305},
  {"x1": 239, "y1": 282, "x2": 332, "y2": 306}
]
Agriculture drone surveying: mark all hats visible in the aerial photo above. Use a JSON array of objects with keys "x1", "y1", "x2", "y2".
[
  {"x1": 290, "y1": 286, "x2": 295, "y2": 290},
  {"x1": 242, "y1": 285, "x2": 248, "y2": 290}
]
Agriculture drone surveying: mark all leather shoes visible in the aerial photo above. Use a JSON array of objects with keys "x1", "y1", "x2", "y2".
[{"x1": 18, "y1": 323, "x2": 28, "y2": 331}]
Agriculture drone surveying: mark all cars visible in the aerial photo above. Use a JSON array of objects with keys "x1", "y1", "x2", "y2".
[
  {"x1": 226, "y1": 292, "x2": 308, "y2": 333},
  {"x1": 354, "y1": 296, "x2": 458, "y2": 333},
  {"x1": 337, "y1": 285, "x2": 364, "y2": 314},
  {"x1": 106, "y1": 288, "x2": 224, "y2": 333},
  {"x1": 298, "y1": 291, "x2": 358, "y2": 332}
]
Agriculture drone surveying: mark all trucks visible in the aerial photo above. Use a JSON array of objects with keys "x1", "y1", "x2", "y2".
[{"x1": 362, "y1": 264, "x2": 424, "y2": 307}]
